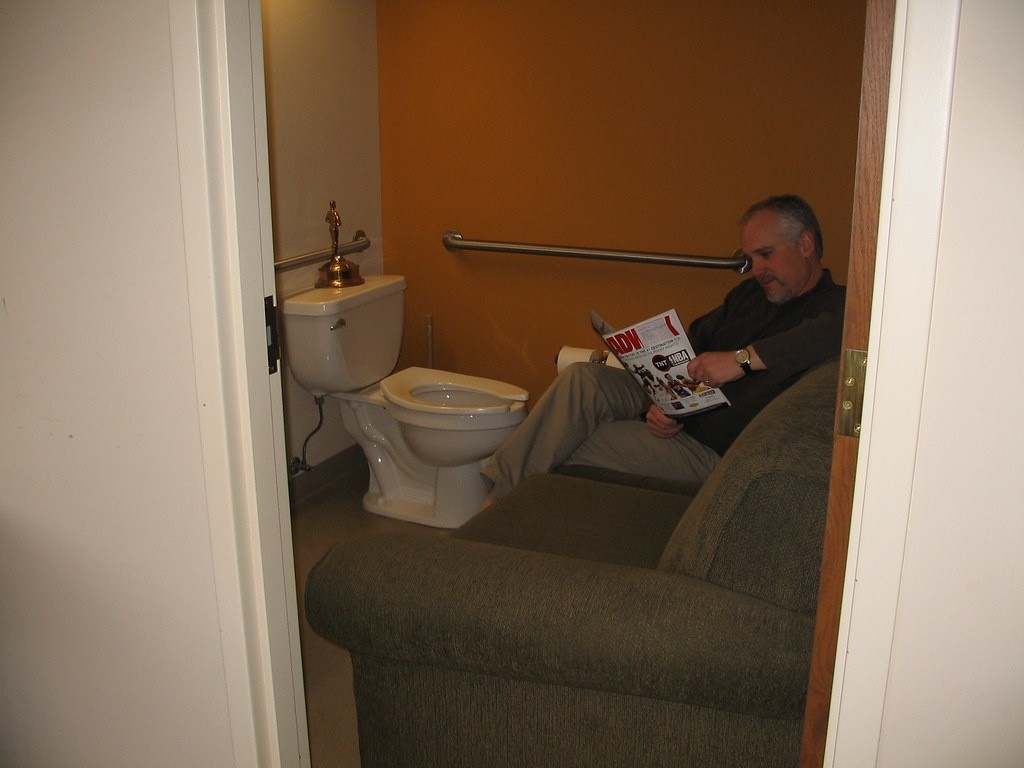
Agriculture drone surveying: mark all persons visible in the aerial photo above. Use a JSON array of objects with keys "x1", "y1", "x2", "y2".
[
  {"x1": 480, "y1": 194, "x2": 847, "y2": 511},
  {"x1": 623, "y1": 361, "x2": 709, "y2": 402}
]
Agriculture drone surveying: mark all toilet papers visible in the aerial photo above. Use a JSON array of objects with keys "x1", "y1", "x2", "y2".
[{"x1": 557, "y1": 346, "x2": 626, "y2": 377}]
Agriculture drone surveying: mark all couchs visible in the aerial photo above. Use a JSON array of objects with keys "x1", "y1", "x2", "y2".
[{"x1": 301, "y1": 353, "x2": 840, "y2": 768}]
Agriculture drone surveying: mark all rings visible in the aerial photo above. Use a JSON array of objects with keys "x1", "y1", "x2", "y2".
[{"x1": 705, "y1": 380, "x2": 710, "y2": 385}]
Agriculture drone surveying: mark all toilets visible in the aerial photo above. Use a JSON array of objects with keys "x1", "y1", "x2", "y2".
[{"x1": 282, "y1": 274, "x2": 531, "y2": 529}]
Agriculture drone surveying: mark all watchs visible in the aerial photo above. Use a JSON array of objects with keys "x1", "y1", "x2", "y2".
[{"x1": 735, "y1": 346, "x2": 755, "y2": 378}]
